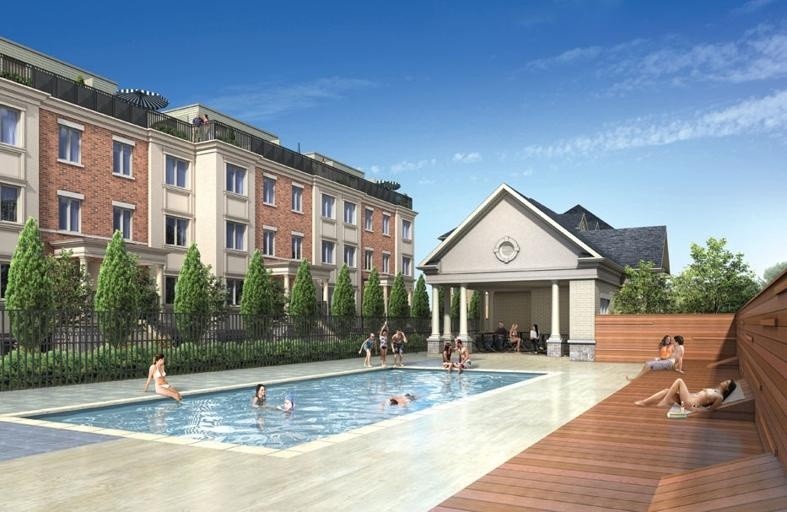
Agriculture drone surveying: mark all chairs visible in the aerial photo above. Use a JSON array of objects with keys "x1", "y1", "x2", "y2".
[{"x1": 474, "y1": 331, "x2": 547, "y2": 354}]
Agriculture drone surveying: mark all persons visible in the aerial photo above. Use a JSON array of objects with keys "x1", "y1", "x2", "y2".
[
  {"x1": 143, "y1": 353, "x2": 182, "y2": 401},
  {"x1": 390, "y1": 330, "x2": 407, "y2": 368},
  {"x1": 453, "y1": 339, "x2": 471, "y2": 374},
  {"x1": 357, "y1": 333, "x2": 375, "y2": 368},
  {"x1": 276, "y1": 393, "x2": 295, "y2": 412},
  {"x1": 495, "y1": 319, "x2": 508, "y2": 335},
  {"x1": 633, "y1": 377, "x2": 736, "y2": 413},
  {"x1": 506, "y1": 322, "x2": 524, "y2": 351},
  {"x1": 625, "y1": 335, "x2": 685, "y2": 381},
  {"x1": 441, "y1": 338, "x2": 458, "y2": 373},
  {"x1": 379, "y1": 393, "x2": 420, "y2": 412},
  {"x1": 378, "y1": 320, "x2": 390, "y2": 367},
  {"x1": 529, "y1": 323, "x2": 544, "y2": 354},
  {"x1": 655, "y1": 335, "x2": 675, "y2": 360},
  {"x1": 251, "y1": 383, "x2": 266, "y2": 408}
]
